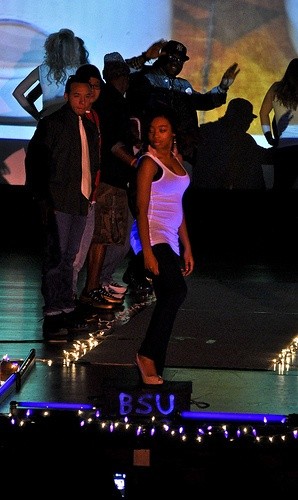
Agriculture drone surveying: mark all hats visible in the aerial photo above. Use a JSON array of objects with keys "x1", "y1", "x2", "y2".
[
  {"x1": 164, "y1": 40, "x2": 189, "y2": 61},
  {"x1": 102, "y1": 52, "x2": 130, "y2": 79},
  {"x1": 228, "y1": 98, "x2": 257, "y2": 118}
]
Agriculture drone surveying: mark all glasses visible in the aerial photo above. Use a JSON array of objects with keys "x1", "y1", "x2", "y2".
[{"x1": 90, "y1": 83, "x2": 102, "y2": 90}]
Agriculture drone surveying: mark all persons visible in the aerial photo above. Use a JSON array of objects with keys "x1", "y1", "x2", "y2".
[
  {"x1": 75, "y1": 40, "x2": 240, "y2": 311},
  {"x1": 261, "y1": 58, "x2": 298, "y2": 148},
  {"x1": 14, "y1": 30, "x2": 81, "y2": 121},
  {"x1": 24, "y1": 78, "x2": 100, "y2": 336},
  {"x1": 129, "y1": 112, "x2": 194, "y2": 385}
]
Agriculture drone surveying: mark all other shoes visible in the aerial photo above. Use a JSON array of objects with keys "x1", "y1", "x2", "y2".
[
  {"x1": 123, "y1": 273, "x2": 153, "y2": 291},
  {"x1": 133, "y1": 354, "x2": 163, "y2": 385},
  {"x1": 42, "y1": 313, "x2": 69, "y2": 338},
  {"x1": 103, "y1": 286, "x2": 125, "y2": 299},
  {"x1": 79, "y1": 289, "x2": 113, "y2": 310},
  {"x1": 107, "y1": 281, "x2": 127, "y2": 294},
  {"x1": 99, "y1": 290, "x2": 124, "y2": 304},
  {"x1": 64, "y1": 311, "x2": 87, "y2": 330}
]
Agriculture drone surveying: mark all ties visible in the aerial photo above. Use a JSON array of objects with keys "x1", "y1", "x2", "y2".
[{"x1": 78, "y1": 115, "x2": 92, "y2": 201}]
